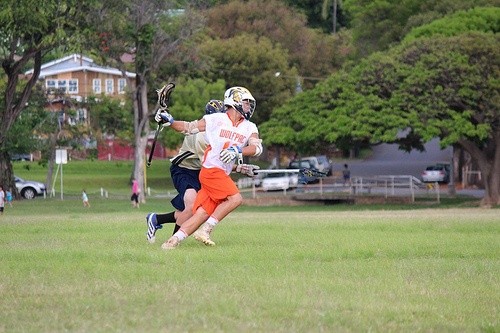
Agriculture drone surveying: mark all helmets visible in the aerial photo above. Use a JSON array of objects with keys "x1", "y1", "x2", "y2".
[
  {"x1": 206, "y1": 100, "x2": 224, "y2": 113},
  {"x1": 224, "y1": 86, "x2": 256, "y2": 119}
]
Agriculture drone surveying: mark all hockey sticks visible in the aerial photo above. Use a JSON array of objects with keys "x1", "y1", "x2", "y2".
[
  {"x1": 253, "y1": 167, "x2": 327, "y2": 177},
  {"x1": 146, "y1": 82, "x2": 176, "y2": 169}
]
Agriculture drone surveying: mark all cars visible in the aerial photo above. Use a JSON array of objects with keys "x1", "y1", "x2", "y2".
[
  {"x1": 261, "y1": 154, "x2": 332, "y2": 192},
  {"x1": 421, "y1": 165, "x2": 449, "y2": 183},
  {"x1": 13, "y1": 175, "x2": 48, "y2": 200}
]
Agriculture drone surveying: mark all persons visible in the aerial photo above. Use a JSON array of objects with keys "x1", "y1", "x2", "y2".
[
  {"x1": 145, "y1": 99, "x2": 256, "y2": 244},
  {"x1": 129, "y1": 178, "x2": 140, "y2": 208},
  {"x1": 5, "y1": 189, "x2": 13, "y2": 208},
  {"x1": 0, "y1": 186, "x2": 5, "y2": 212},
  {"x1": 81, "y1": 190, "x2": 91, "y2": 208},
  {"x1": 154, "y1": 85, "x2": 264, "y2": 248},
  {"x1": 342, "y1": 163, "x2": 352, "y2": 187}
]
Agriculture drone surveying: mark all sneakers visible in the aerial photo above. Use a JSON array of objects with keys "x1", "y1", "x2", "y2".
[
  {"x1": 147, "y1": 213, "x2": 162, "y2": 243},
  {"x1": 193, "y1": 222, "x2": 216, "y2": 247},
  {"x1": 162, "y1": 235, "x2": 180, "y2": 250}
]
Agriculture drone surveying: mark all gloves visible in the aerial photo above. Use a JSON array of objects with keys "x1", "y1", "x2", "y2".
[
  {"x1": 235, "y1": 164, "x2": 260, "y2": 177},
  {"x1": 154, "y1": 109, "x2": 173, "y2": 127},
  {"x1": 219, "y1": 145, "x2": 237, "y2": 163}
]
